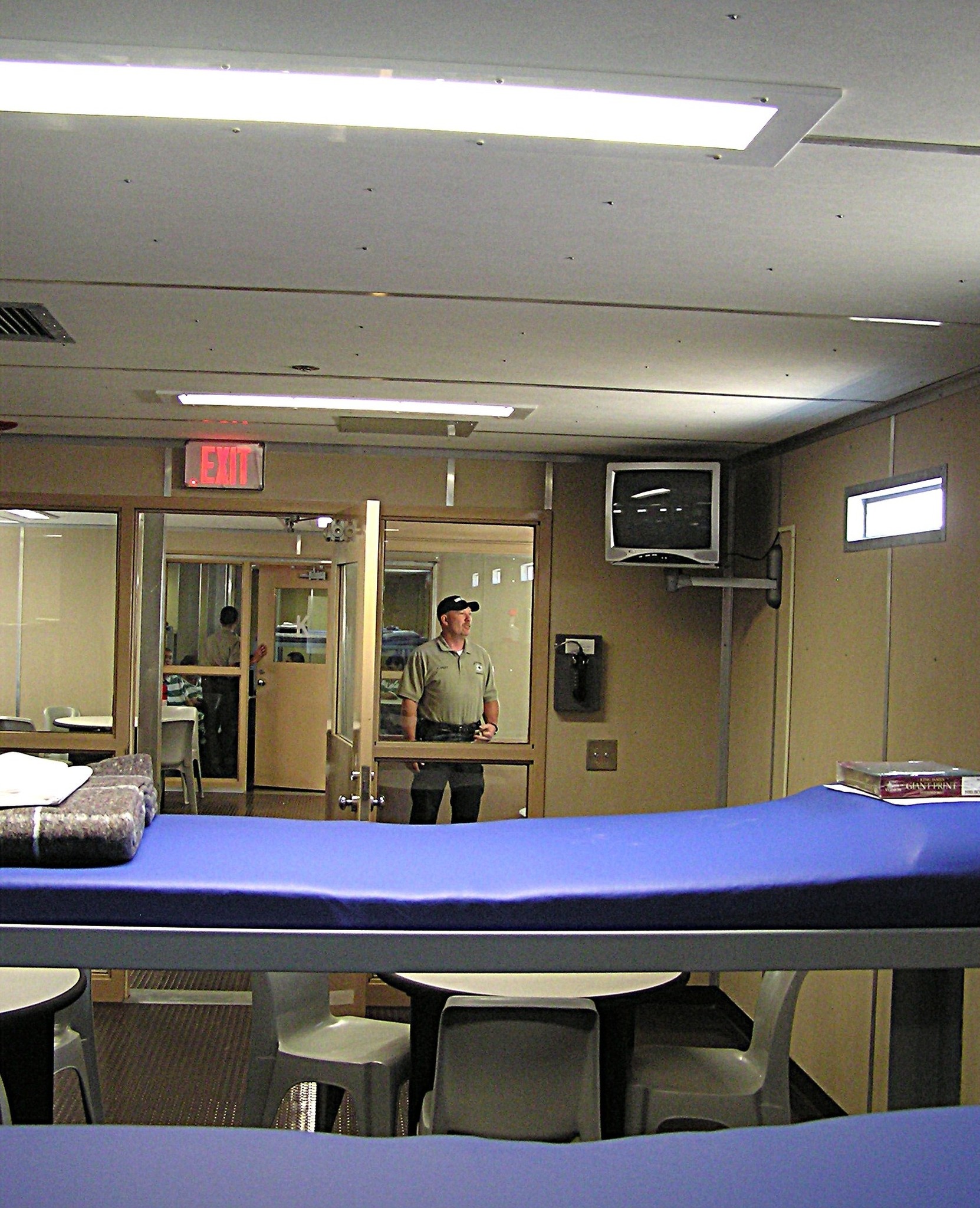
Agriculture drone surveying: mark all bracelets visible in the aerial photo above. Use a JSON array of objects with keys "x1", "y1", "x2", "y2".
[{"x1": 486, "y1": 723, "x2": 498, "y2": 735}]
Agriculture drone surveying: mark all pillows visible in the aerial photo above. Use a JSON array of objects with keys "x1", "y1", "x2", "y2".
[{"x1": 392, "y1": 630, "x2": 421, "y2": 639}]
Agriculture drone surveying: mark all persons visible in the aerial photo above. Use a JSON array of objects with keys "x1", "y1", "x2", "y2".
[
  {"x1": 379, "y1": 656, "x2": 404, "y2": 714},
  {"x1": 286, "y1": 652, "x2": 305, "y2": 663},
  {"x1": 396, "y1": 595, "x2": 500, "y2": 825},
  {"x1": 201, "y1": 606, "x2": 267, "y2": 778},
  {"x1": 163, "y1": 648, "x2": 206, "y2": 748}
]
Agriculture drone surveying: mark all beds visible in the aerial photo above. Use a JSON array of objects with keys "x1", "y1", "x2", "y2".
[
  {"x1": 274, "y1": 630, "x2": 430, "y2": 662},
  {"x1": 0, "y1": 1102, "x2": 980, "y2": 1208},
  {"x1": 0, "y1": 780, "x2": 980, "y2": 1115}
]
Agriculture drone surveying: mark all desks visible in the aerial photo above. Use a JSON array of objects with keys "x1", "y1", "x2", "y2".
[
  {"x1": 0, "y1": 967, "x2": 88, "y2": 1126},
  {"x1": 380, "y1": 696, "x2": 403, "y2": 715},
  {"x1": 53, "y1": 715, "x2": 139, "y2": 766},
  {"x1": 378, "y1": 972, "x2": 692, "y2": 1144}
]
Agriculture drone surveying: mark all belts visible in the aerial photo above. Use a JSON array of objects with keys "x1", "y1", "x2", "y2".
[{"x1": 422, "y1": 720, "x2": 481, "y2": 733}]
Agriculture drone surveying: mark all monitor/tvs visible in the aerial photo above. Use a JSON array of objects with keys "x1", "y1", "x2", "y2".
[{"x1": 604, "y1": 461, "x2": 720, "y2": 564}]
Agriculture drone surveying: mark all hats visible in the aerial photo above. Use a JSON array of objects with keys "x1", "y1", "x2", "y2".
[{"x1": 437, "y1": 595, "x2": 479, "y2": 621}]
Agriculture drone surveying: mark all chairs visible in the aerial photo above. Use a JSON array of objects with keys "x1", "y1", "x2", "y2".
[
  {"x1": 0, "y1": 716, "x2": 40, "y2": 758},
  {"x1": 160, "y1": 719, "x2": 198, "y2": 815},
  {"x1": 415, "y1": 995, "x2": 606, "y2": 1142},
  {"x1": 43, "y1": 706, "x2": 81, "y2": 760},
  {"x1": 623, "y1": 970, "x2": 807, "y2": 1138},
  {"x1": 239, "y1": 972, "x2": 410, "y2": 1140},
  {"x1": 161, "y1": 705, "x2": 204, "y2": 805},
  {"x1": 52, "y1": 966, "x2": 104, "y2": 1125}
]
